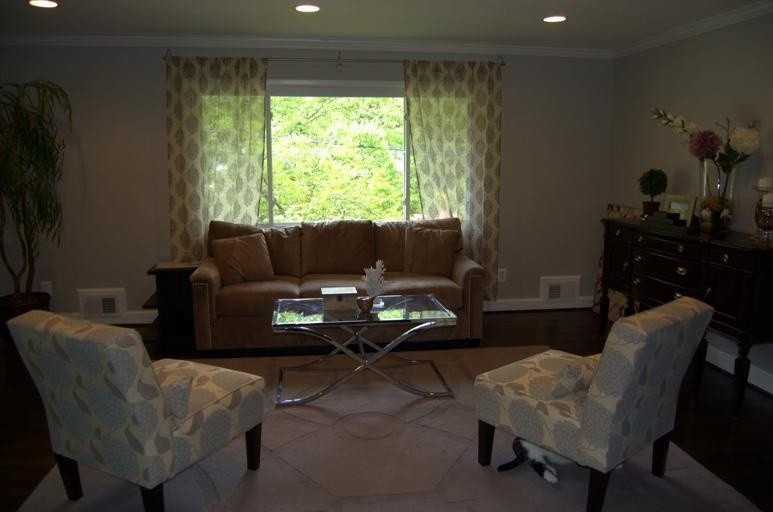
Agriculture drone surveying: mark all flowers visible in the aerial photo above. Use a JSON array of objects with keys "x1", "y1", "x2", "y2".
[{"x1": 648, "y1": 100, "x2": 761, "y2": 227}]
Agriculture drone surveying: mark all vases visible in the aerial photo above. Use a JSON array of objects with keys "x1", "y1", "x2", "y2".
[{"x1": 699, "y1": 154, "x2": 738, "y2": 242}]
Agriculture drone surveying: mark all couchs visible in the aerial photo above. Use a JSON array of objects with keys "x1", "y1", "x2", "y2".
[{"x1": 188, "y1": 216, "x2": 489, "y2": 359}]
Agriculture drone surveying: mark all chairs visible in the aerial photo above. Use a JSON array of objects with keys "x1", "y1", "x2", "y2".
[
  {"x1": 5, "y1": 307, "x2": 267, "y2": 510},
  {"x1": 474, "y1": 296, "x2": 718, "y2": 512}
]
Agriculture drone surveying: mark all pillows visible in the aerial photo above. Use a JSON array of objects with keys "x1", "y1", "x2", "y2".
[
  {"x1": 210, "y1": 232, "x2": 276, "y2": 287},
  {"x1": 403, "y1": 226, "x2": 459, "y2": 281}
]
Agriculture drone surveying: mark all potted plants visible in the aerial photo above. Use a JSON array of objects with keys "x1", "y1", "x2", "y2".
[
  {"x1": 638, "y1": 166, "x2": 669, "y2": 219},
  {"x1": 0, "y1": 80, "x2": 73, "y2": 354}
]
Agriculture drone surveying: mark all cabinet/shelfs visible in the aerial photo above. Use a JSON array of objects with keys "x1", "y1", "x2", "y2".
[{"x1": 601, "y1": 215, "x2": 773, "y2": 417}]
